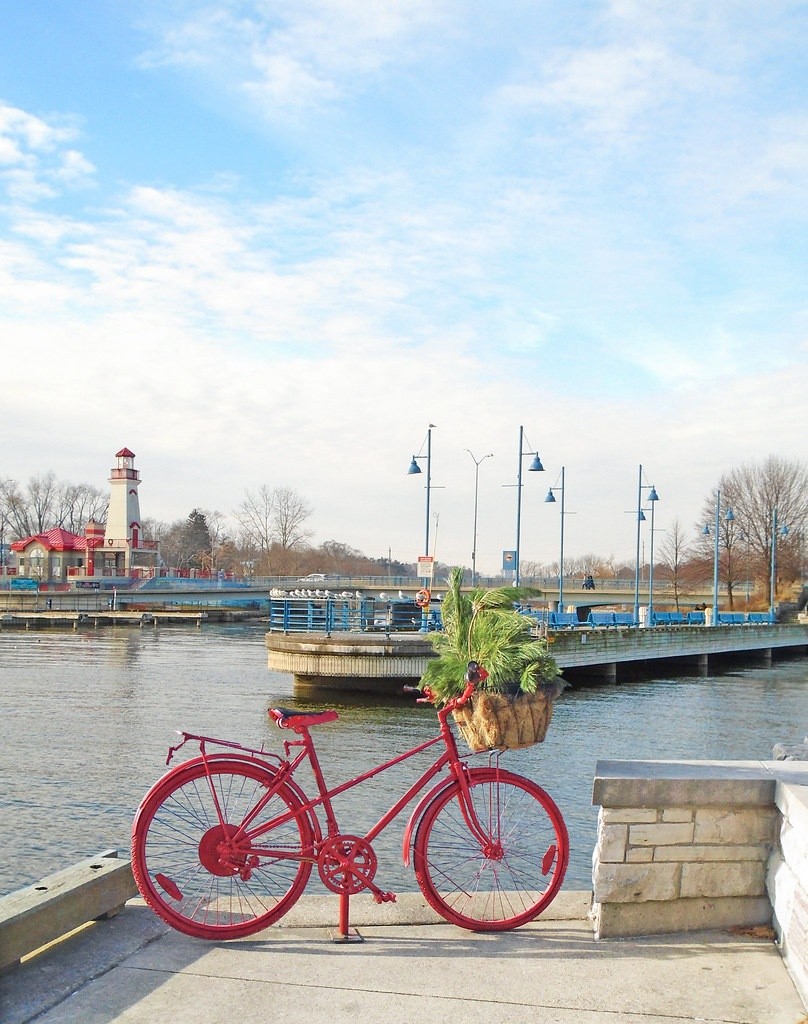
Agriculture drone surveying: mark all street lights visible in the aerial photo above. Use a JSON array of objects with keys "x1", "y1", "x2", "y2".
[
  {"x1": 769, "y1": 509, "x2": 788, "y2": 623},
  {"x1": 408, "y1": 428, "x2": 445, "y2": 632},
  {"x1": 624, "y1": 464, "x2": 660, "y2": 628},
  {"x1": 502, "y1": 425, "x2": 545, "y2": 587},
  {"x1": 544, "y1": 466, "x2": 577, "y2": 614},
  {"x1": 636, "y1": 501, "x2": 666, "y2": 625},
  {"x1": 702, "y1": 490, "x2": 735, "y2": 626},
  {"x1": 464, "y1": 449, "x2": 493, "y2": 587}
]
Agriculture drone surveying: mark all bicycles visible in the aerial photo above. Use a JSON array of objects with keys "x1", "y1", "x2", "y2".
[{"x1": 131, "y1": 661, "x2": 569, "y2": 942}]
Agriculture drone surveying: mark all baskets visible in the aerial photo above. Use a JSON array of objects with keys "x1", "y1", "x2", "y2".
[{"x1": 452, "y1": 681, "x2": 552, "y2": 749}]
